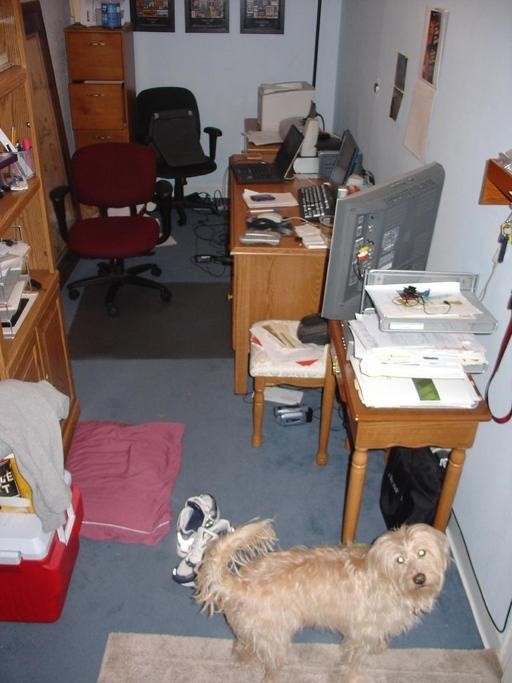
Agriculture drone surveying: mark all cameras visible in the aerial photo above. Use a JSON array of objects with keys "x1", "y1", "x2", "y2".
[{"x1": 275, "y1": 405, "x2": 314, "y2": 427}]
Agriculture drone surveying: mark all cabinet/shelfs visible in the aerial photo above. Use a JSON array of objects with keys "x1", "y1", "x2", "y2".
[
  {"x1": 61, "y1": 21, "x2": 136, "y2": 220},
  {"x1": 0, "y1": 0, "x2": 82, "y2": 466}
]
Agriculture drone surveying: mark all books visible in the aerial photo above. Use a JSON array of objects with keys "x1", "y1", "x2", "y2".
[
  {"x1": 0, "y1": 239, "x2": 39, "y2": 338},
  {"x1": 69, "y1": 0, "x2": 124, "y2": 27}
]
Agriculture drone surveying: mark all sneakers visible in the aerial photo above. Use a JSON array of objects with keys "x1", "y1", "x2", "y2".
[
  {"x1": 172, "y1": 519, "x2": 234, "y2": 587},
  {"x1": 176, "y1": 493, "x2": 219, "y2": 558}
]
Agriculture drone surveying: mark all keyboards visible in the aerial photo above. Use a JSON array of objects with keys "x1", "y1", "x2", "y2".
[{"x1": 299, "y1": 183, "x2": 324, "y2": 224}]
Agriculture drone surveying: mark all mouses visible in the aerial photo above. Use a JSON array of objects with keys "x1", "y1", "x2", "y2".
[{"x1": 257, "y1": 213, "x2": 283, "y2": 222}]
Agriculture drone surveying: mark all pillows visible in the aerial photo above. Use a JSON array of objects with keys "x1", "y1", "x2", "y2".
[{"x1": 65, "y1": 421, "x2": 184, "y2": 546}]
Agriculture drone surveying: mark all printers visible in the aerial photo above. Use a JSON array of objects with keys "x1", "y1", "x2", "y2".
[{"x1": 258, "y1": 82, "x2": 317, "y2": 132}]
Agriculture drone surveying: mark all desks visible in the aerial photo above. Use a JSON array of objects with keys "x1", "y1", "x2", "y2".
[
  {"x1": 316, "y1": 318, "x2": 493, "y2": 546},
  {"x1": 229, "y1": 149, "x2": 345, "y2": 396},
  {"x1": 242, "y1": 116, "x2": 291, "y2": 155}
]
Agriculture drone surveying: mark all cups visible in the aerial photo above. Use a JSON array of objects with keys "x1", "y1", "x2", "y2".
[{"x1": 319, "y1": 215, "x2": 335, "y2": 238}]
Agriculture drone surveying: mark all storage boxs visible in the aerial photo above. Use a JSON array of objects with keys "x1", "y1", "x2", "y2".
[{"x1": 0, "y1": 483, "x2": 83, "y2": 624}]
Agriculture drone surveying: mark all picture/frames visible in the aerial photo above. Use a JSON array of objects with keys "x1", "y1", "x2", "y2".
[
  {"x1": 240, "y1": 0, "x2": 284, "y2": 34},
  {"x1": 130, "y1": 0, "x2": 174, "y2": 32},
  {"x1": 184, "y1": 0, "x2": 229, "y2": 33}
]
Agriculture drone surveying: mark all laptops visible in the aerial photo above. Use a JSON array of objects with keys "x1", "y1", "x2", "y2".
[{"x1": 232, "y1": 125, "x2": 305, "y2": 185}]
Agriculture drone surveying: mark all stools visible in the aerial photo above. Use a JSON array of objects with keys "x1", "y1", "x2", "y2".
[{"x1": 249, "y1": 317, "x2": 333, "y2": 450}]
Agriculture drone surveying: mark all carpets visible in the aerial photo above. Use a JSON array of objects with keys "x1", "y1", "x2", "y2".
[{"x1": 94, "y1": 631, "x2": 506, "y2": 683}]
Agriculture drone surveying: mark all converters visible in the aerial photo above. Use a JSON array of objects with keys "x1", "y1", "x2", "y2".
[{"x1": 195, "y1": 253, "x2": 212, "y2": 263}]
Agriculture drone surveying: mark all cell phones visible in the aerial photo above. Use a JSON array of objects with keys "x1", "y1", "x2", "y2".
[{"x1": 252, "y1": 193, "x2": 273, "y2": 202}]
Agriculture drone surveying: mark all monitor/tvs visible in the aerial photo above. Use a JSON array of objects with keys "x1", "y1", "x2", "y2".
[
  {"x1": 322, "y1": 162, "x2": 446, "y2": 322},
  {"x1": 331, "y1": 132, "x2": 359, "y2": 188}
]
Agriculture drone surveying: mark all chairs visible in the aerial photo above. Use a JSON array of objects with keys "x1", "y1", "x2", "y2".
[
  {"x1": 134, "y1": 86, "x2": 222, "y2": 224},
  {"x1": 48, "y1": 142, "x2": 173, "y2": 314}
]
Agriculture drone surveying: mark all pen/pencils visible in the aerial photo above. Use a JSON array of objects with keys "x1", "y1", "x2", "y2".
[
  {"x1": 11, "y1": 125, "x2": 34, "y2": 171},
  {"x1": 6, "y1": 144, "x2": 27, "y2": 177}
]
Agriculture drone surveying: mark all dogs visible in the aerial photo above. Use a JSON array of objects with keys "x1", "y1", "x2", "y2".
[{"x1": 188, "y1": 513, "x2": 451, "y2": 679}]
